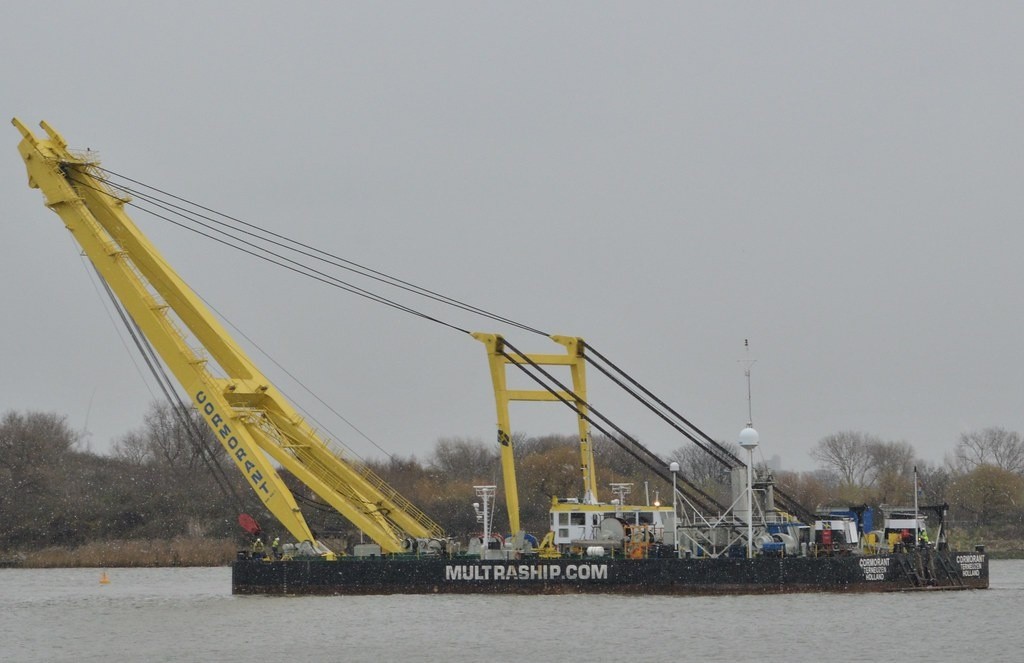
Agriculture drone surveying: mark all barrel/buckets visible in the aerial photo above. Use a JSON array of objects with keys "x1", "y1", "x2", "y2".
[{"x1": 587, "y1": 546, "x2": 605, "y2": 557}]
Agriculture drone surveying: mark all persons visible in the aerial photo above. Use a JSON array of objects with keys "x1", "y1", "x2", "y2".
[
  {"x1": 919, "y1": 528, "x2": 927, "y2": 548},
  {"x1": 254, "y1": 538, "x2": 266, "y2": 560},
  {"x1": 271, "y1": 537, "x2": 281, "y2": 560}
]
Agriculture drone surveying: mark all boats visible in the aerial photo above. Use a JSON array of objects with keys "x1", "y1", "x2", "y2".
[{"x1": 10, "y1": 117, "x2": 991, "y2": 598}]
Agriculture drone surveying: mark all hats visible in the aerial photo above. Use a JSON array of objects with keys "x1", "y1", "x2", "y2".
[
  {"x1": 257, "y1": 538, "x2": 260, "y2": 541},
  {"x1": 275, "y1": 538, "x2": 279, "y2": 540}
]
